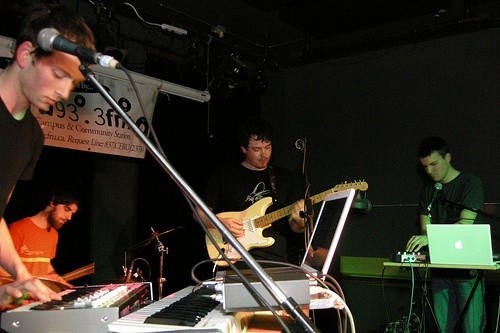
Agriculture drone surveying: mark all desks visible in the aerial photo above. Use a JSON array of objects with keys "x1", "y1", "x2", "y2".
[{"x1": 382, "y1": 262, "x2": 500, "y2": 333}]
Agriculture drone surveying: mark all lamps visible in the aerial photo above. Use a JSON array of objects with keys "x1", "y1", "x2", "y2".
[{"x1": 352, "y1": 191, "x2": 368, "y2": 211}]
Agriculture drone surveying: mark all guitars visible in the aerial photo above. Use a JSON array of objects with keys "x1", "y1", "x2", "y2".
[{"x1": 205, "y1": 179, "x2": 369, "y2": 267}]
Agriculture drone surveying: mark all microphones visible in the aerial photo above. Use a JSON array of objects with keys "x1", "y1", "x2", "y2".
[
  {"x1": 427, "y1": 182, "x2": 442, "y2": 212},
  {"x1": 37, "y1": 27, "x2": 122, "y2": 70},
  {"x1": 126, "y1": 262, "x2": 134, "y2": 282},
  {"x1": 295, "y1": 139, "x2": 305, "y2": 150}
]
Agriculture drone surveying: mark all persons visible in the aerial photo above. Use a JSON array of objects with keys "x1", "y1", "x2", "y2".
[
  {"x1": 193, "y1": 119, "x2": 308, "y2": 272},
  {"x1": 0, "y1": 7, "x2": 96, "y2": 310},
  {"x1": 0, "y1": 190, "x2": 82, "y2": 310},
  {"x1": 406, "y1": 136, "x2": 486, "y2": 333}
]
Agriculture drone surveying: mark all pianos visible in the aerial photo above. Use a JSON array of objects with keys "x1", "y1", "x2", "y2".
[
  {"x1": 107, "y1": 280, "x2": 310, "y2": 333},
  {"x1": 0, "y1": 281, "x2": 155, "y2": 333}
]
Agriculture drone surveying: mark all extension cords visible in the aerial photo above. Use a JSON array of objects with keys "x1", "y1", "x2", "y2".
[{"x1": 161, "y1": 24, "x2": 187, "y2": 36}]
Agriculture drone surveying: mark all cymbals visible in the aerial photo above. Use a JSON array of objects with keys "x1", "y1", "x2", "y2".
[{"x1": 123, "y1": 225, "x2": 185, "y2": 252}]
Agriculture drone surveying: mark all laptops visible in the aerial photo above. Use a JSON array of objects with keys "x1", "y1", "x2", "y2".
[{"x1": 426, "y1": 224, "x2": 500, "y2": 266}]
